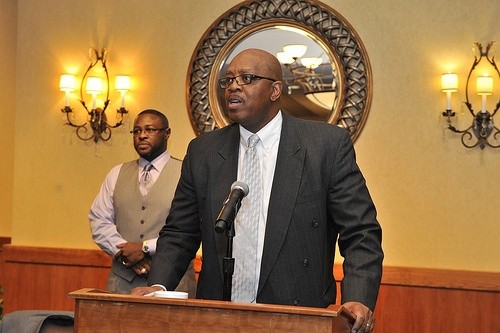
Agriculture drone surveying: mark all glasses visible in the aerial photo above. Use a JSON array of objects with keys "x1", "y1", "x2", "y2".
[
  {"x1": 130, "y1": 128, "x2": 167, "y2": 137},
  {"x1": 218, "y1": 74, "x2": 277, "y2": 89}
]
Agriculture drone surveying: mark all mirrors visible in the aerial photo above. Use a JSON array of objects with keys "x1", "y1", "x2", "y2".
[{"x1": 185, "y1": 0, "x2": 373, "y2": 145}]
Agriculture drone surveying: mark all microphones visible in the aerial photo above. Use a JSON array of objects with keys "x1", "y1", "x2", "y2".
[{"x1": 213, "y1": 181, "x2": 249, "y2": 234}]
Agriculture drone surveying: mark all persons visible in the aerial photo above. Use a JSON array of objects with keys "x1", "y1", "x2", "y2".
[
  {"x1": 131, "y1": 48, "x2": 384, "y2": 333},
  {"x1": 88, "y1": 109, "x2": 196, "y2": 297}
]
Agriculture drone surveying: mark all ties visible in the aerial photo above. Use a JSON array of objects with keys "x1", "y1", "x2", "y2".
[
  {"x1": 230, "y1": 134, "x2": 260, "y2": 305},
  {"x1": 138, "y1": 163, "x2": 154, "y2": 198}
]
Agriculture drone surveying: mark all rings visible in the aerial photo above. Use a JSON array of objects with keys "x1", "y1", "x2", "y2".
[
  {"x1": 123, "y1": 261, "x2": 126, "y2": 264},
  {"x1": 141, "y1": 268, "x2": 146, "y2": 273},
  {"x1": 366, "y1": 324, "x2": 370, "y2": 327}
]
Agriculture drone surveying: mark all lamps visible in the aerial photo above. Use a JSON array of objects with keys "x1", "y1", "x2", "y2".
[
  {"x1": 440, "y1": 41, "x2": 500, "y2": 150},
  {"x1": 277, "y1": 44, "x2": 337, "y2": 112},
  {"x1": 58, "y1": 48, "x2": 134, "y2": 145}
]
